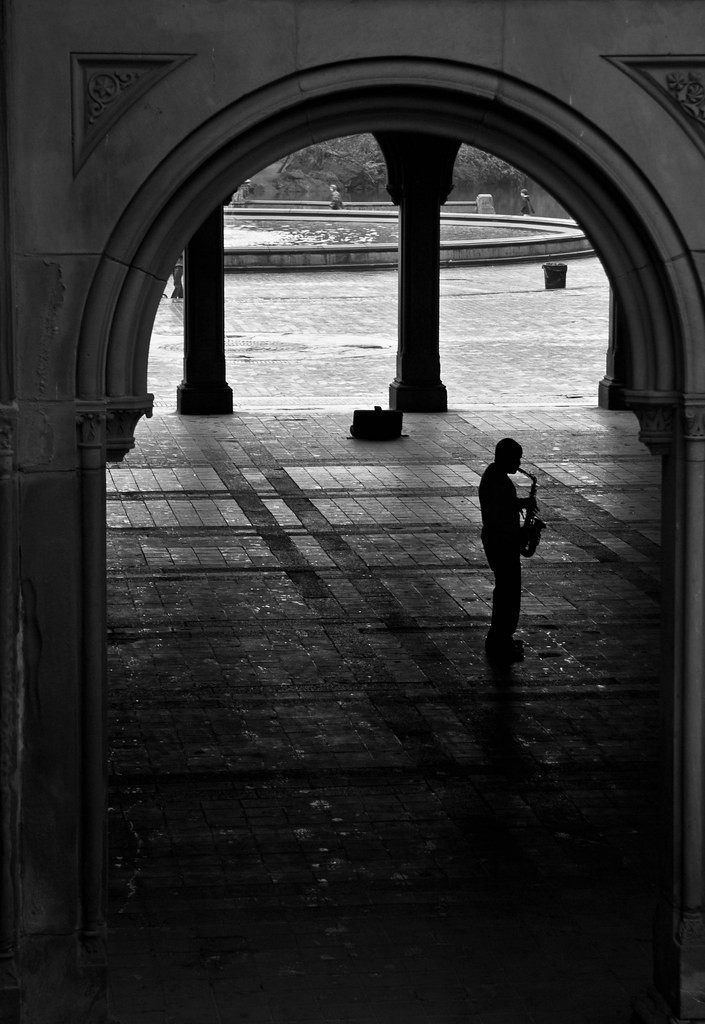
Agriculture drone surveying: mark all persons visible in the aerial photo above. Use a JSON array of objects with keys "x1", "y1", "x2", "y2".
[
  {"x1": 478, "y1": 438, "x2": 541, "y2": 666},
  {"x1": 171, "y1": 250, "x2": 183, "y2": 298},
  {"x1": 328, "y1": 184, "x2": 344, "y2": 210},
  {"x1": 520, "y1": 189, "x2": 535, "y2": 215}
]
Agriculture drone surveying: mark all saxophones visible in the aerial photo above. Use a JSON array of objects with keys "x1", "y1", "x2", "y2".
[{"x1": 514, "y1": 468, "x2": 547, "y2": 558}]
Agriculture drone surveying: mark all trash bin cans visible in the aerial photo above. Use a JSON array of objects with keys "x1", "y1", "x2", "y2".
[{"x1": 542, "y1": 262, "x2": 568, "y2": 289}]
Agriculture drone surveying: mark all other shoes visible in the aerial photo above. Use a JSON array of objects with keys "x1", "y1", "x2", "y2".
[{"x1": 485, "y1": 638, "x2": 524, "y2": 662}]
